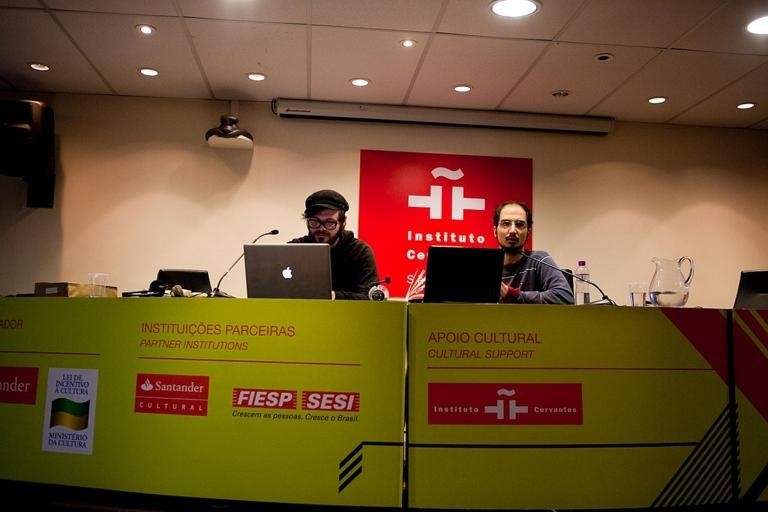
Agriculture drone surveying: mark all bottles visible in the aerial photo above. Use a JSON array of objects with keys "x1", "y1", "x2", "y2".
[{"x1": 573, "y1": 259, "x2": 591, "y2": 305}]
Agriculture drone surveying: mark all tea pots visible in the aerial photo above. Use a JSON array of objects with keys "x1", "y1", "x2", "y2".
[{"x1": 648, "y1": 255, "x2": 696, "y2": 307}]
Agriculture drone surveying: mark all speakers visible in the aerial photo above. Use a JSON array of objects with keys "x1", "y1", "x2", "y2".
[{"x1": 0, "y1": 99, "x2": 55, "y2": 178}]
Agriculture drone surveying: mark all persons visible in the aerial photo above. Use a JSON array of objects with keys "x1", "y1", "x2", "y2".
[
  {"x1": 285, "y1": 189, "x2": 379, "y2": 300},
  {"x1": 491, "y1": 199, "x2": 575, "y2": 304}
]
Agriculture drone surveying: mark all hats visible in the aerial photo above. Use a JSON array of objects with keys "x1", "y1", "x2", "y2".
[{"x1": 305, "y1": 190, "x2": 349, "y2": 212}]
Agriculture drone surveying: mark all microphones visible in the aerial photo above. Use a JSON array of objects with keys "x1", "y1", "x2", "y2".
[
  {"x1": 517, "y1": 249, "x2": 617, "y2": 305},
  {"x1": 208, "y1": 230, "x2": 278, "y2": 298},
  {"x1": 370, "y1": 277, "x2": 391, "y2": 285}
]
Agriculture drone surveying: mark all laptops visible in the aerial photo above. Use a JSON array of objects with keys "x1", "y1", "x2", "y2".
[
  {"x1": 409, "y1": 246, "x2": 505, "y2": 303},
  {"x1": 244, "y1": 243, "x2": 332, "y2": 298},
  {"x1": 732, "y1": 270, "x2": 768, "y2": 310}
]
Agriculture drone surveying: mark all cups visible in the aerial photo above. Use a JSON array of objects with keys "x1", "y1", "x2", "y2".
[
  {"x1": 87, "y1": 272, "x2": 109, "y2": 298},
  {"x1": 628, "y1": 282, "x2": 649, "y2": 307}
]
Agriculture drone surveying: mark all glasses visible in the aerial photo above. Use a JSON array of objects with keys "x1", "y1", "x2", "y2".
[
  {"x1": 308, "y1": 218, "x2": 337, "y2": 230},
  {"x1": 500, "y1": 220, "x2": 527, "y2": 230}
]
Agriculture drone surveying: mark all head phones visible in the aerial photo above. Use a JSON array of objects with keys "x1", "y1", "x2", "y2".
[{"x1": 122, "y1": 280, "x2": 167, "y2": 297}]
